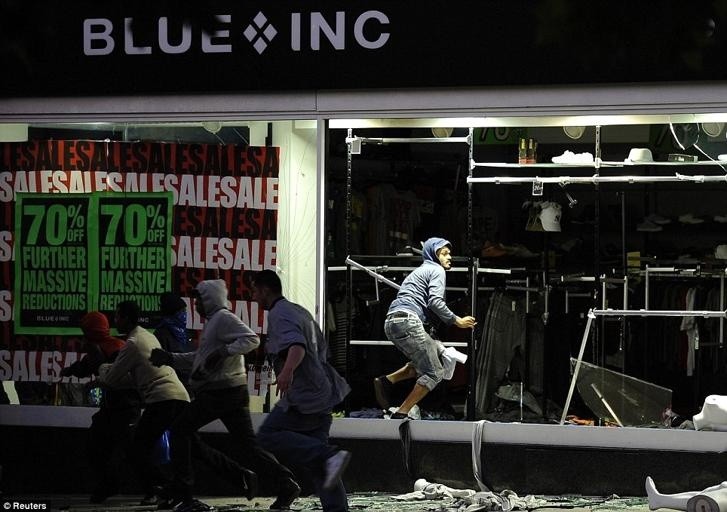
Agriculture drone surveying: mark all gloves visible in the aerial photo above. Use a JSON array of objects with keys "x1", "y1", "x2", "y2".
[
  {"x1": 150, "y1": 348, "x2": 172, "y2": 367},
  {"x1": 204, "y1": 346, "x2": 229, "y2": 370}
]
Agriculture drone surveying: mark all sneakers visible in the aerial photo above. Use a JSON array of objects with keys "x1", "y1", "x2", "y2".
[
  {"x1": 373, "y1": 375, "x2": 393, "y2": 410},
  {"x1": 495, "y1": 243, "x2": 520, "y2": 256},
  {"x1": 321, "y1": 449, "x2": 352, "y2": 489},
  {"x1": 643, "y1": 212, "x2": 671, "y2": 224},
  {"x1": 512, "y1": 243, "x2": 540, "y2": 257},
  {"x1": 243, "y1": 468, "x2": 258, "y2": 501},
  {"x1": 157, "y1": 498, "x2": 193, "y2": 511},
  {"x1": 391, "y1": 412, "x2": 415, "y2": 420},
  {"x1": 636, "y1": 222, "x2": 663, "y2": 231},
  {"x1": 140, "y1": 495, "x2": 159, "y2": 507},
  {"x1": 270, "y1": 478, "x2": 301, "y2": 510},
  {"x1": 551, "y1": 150, "x2": 593, "y2": 164},
  {"x1": 679, "y1": 213, "x2": 704, "y2": 223},
  {"x1": 480, "y1": 240, "x2": 506, "y2": 257}
]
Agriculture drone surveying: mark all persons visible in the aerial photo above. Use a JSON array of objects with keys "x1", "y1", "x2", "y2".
[
  {"x1": 644, "y1": 475, "x2": 727, "y2": 511},
  {"x1": 373, "y1": 236, "x2": 478, "y2": 419},
  {"x1": 58, "y1": 269, "x2": 353, "y2": 511}
]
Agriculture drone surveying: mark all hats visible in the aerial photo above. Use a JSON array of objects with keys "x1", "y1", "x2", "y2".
[
  {"x1": 160, "y1": 292, "x2": 187, "y2": 315},
  {"x1": 524, "y1": 207, "x2": 544, "y2": 232},
  {"x1": 538, "y1": 206, "x2": 562, "y2": 232},
  {"x1": 81, "y1": 311, "x2": 110, "y2": 336},
  {"x1": 624, "y1": 148, "x2": 656, "y2": 162}
]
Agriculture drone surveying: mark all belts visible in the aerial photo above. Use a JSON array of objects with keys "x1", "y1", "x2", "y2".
[{"x1": 386, "y1": 311, "x2": 410, "y2": 319}]
